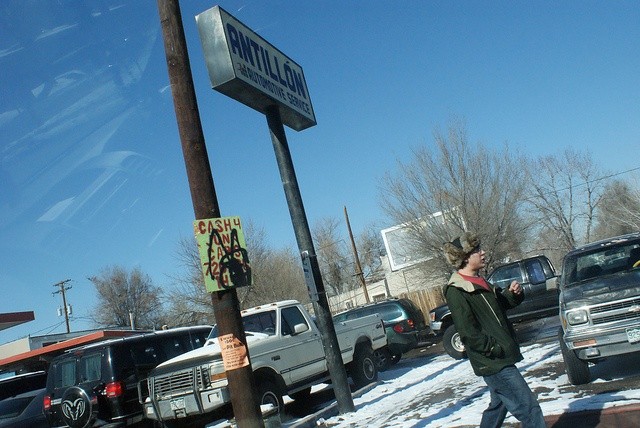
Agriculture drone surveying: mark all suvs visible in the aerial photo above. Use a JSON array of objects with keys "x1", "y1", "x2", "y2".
[
  {"x1": 558, "y1": 233, "x2": 640, "y2": 384},
  {"x1": 332, "y1": 297, "x2": 430, "y2": 363}
]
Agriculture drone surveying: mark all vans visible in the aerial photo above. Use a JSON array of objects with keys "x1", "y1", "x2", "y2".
[{"x1": 43, "y1": 325, "x2": 214, "y2": 427}]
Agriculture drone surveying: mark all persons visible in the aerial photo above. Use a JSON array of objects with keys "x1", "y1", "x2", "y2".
[{"x1": 442, "y1": 231, "x2": 547, "y2": 428}]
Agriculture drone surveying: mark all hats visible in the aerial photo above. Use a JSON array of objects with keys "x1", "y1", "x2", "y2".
[{"x1": 443, "y1": 230, "x2": 481, "y2": 268}]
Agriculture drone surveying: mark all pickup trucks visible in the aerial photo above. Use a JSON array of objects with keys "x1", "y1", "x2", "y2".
[
  {"x1": 137, "y1": 299, "x2": 389, "y2": 422},
  {"x1": 429, "y1": 256, "x2": 562, "y2": 360}
]
